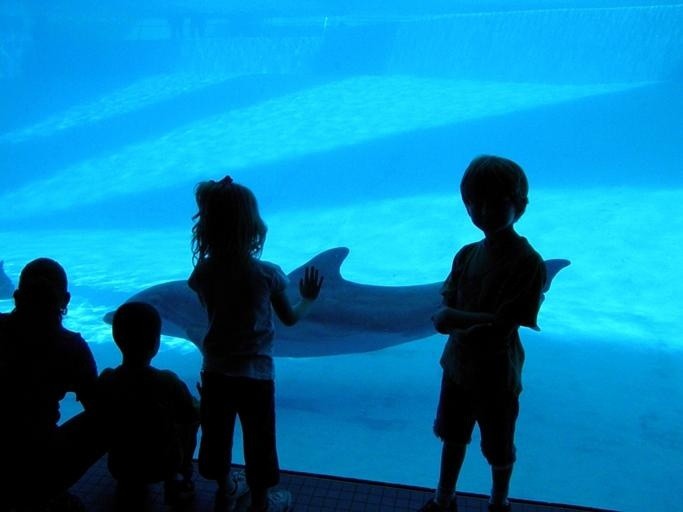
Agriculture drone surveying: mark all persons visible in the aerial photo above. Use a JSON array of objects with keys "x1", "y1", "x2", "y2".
[
  {"x1": 0, "y1": 255, "x2": 123, "y2": 510},
  {"x1": 182, "y1": 174, "x2": 326, "y2": 511},
  {"x1": 84, "y1": 299, "x2": 208, "y2": 504},
  {"x1": 419, "y1": 150, "x2": 548, "y2": 512}
]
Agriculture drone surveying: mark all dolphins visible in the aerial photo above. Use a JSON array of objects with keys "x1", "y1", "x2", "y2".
[{"x1": 103, "y1": 248, "x2": 571, "y2": 358}]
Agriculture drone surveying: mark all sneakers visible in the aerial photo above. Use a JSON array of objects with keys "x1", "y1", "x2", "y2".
[
  {"x1": 214, "y1": 467, "x2": 251, "y2": 512},
  {"x1": 248, "y1": 490, "x2": 293, "y2": 512},
  {"x1": 163, "y1": 481, "x2": 195, "y2": 506},
  {"x1": 416, "y1": 495, "x2": 459, "y2": 512}
]
